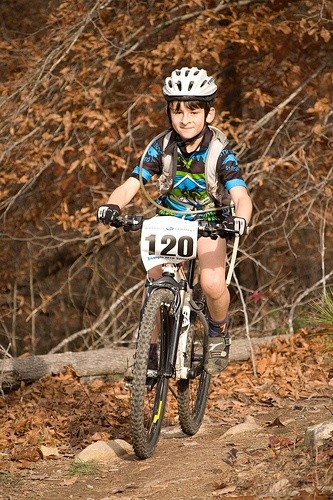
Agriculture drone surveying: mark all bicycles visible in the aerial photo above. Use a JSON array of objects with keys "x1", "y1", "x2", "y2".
[{"x1": 99, "y1": 215, "x2": 250, "y2": 460}]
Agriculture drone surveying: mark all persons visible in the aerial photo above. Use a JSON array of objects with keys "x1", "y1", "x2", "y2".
[{"x1": 97, "y1": 67, "x2": 253, "y2": 381}]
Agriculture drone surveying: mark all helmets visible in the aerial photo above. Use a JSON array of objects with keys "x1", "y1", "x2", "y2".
[{"x1": 162, "y1": 66, "x2": 218, "y2": 101}]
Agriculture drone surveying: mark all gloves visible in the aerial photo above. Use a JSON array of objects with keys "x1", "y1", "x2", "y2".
[
  {"x1": 96, "y1": 204, "x2": 121, "y2": 226},
  {"x1": 223, "y1": 216, "x2": 246, "y2": 237}
]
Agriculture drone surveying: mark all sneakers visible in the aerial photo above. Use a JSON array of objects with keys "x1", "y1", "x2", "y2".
[
  {"x1": 125, "y1": 357, "x2": 159, "y2": 392},
  {"x1": 203, "y1": 332, "x2": 231, "y2": 376}
]
